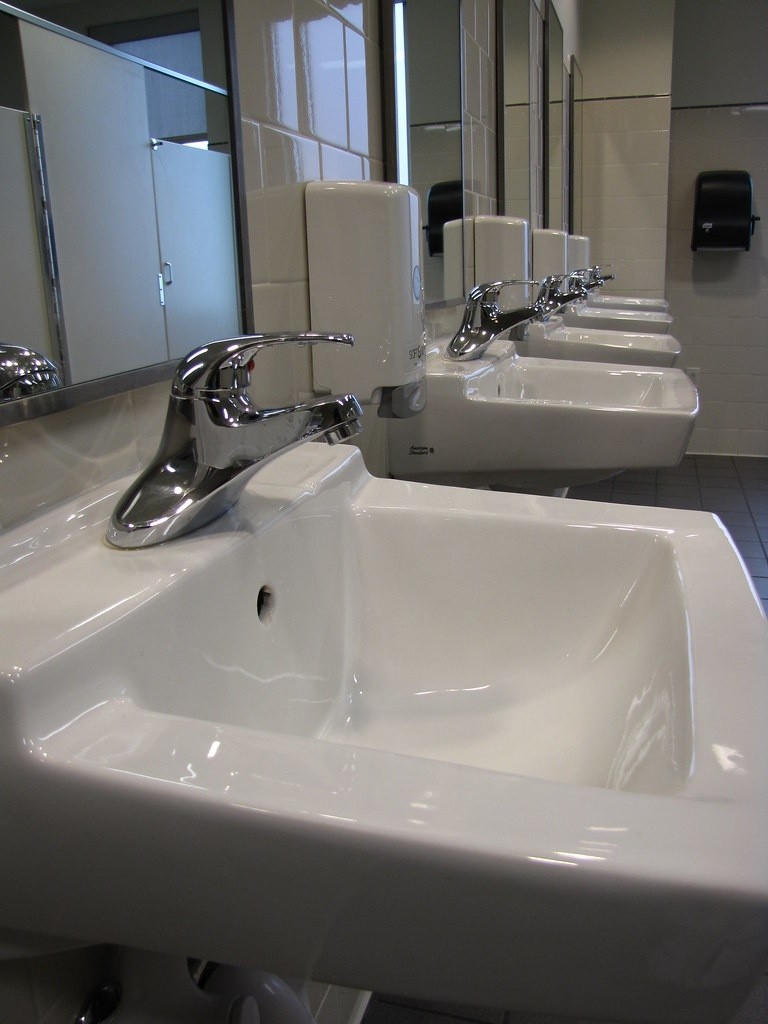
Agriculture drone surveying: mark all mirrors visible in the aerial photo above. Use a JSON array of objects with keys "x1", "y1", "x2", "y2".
[
  {"x1": 0, "y1": 3, "x2": 249, "y2": 427},
  {"x1": 378, "y1": 0, "x2": 585, "y2": 313}
]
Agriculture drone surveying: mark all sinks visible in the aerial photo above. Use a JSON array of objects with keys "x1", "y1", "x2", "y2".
[
  {"x1": 129, "y1": 508, "x2": 768, "y2": 1024},
  {"x1": 598, "y1": 296, "x2": 669, "y2": 310},
  {"x1": 556, "y1": 328, "x2": 681, "y2": 368},
  {"x1": 469, "y1": 354, "x2": 699, "y2": 474},
  {"x1": 583, "y1": 307, "x2": 674, "y2": 332}
]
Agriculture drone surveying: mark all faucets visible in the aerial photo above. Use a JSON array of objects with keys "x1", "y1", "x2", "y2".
[
  {"x1": 109, "y1": 330, "x2": 366, "y2": 549},
  {"x1": 1, "y1": 346, "x2": 66, "y2": 405},
  {"x1": 569, "y1": 269, "x2": 605, "y2": 290},
  {"x1": 536, "y1": 275, "x2": 585, "y2": 321},
  {"x1": 590, "y1": 264, "x2": 616, "y2": 282},
  {"x1": 448, "y1": 279, "x2": 544, "y2": 361}
]
